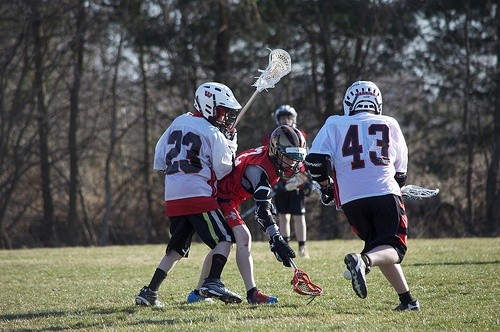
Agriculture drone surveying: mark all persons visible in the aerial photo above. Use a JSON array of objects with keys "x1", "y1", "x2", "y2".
[
  {"x1": 186, "y1": 126, "x2": 307, "y2": 305},
  {"x1": 266, "y1": 105, "x2": 309, "y2": 260},
  {"x1": 305, "y1": 81, "x2": 421, "y2": 311},
  {"x1": 135, "y1": 82, "x2": 242, "y2": 311}
]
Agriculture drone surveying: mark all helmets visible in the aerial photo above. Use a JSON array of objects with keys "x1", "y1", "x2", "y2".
[
  {"x1": 268, "y1": 124, "x2": 306, "y2": 160},
  {"x1": 194, "y1": 82, "x2": 242, "y2": 123},
  {"x1": 275, "y1": 105, "x2": 298, "y2": 126},
  {"x1": 344, "y1": 80, "x2": 383, "y2": 116}
]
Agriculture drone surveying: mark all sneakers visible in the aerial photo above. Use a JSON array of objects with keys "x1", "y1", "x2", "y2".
[
  {"x1": 135, "y1": 285, "x2": 163, "y2": 308},
  {"x1": 187, "y1": 291, "x2": 213, "y2": 304},
  {"x1": 394, "y1": 299, "x2": 419, "y2": 311},
  {"x1": 344, "y1": 252, "x2": 368, "y2": 299},
  {"x1": 198, "y1": 277, "x2": 242, "y2": 304},
  {"x1": 247, "y1": 290, "x2": 278, "y2": 304}
]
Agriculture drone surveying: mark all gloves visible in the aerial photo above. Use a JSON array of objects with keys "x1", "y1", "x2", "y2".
[
  {"x1": 225, "y1": 127, "x2": 238, "y2": 146},
  {"x1": 269, "y1": 204, "x2": 277, "y2": 219},
  {"x1": 269, "y1": 233, "x2": 296, "y2": 267},
  {"x1": 318, "y1": 176, "x2": 338, "y2": 206}
]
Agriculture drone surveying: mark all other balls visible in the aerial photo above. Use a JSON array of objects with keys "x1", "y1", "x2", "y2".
[{"x1": 343, "y1": 269, "x2": 352, "y2": 280}]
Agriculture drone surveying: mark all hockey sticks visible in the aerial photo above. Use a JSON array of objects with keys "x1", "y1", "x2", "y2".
[
  {"x1": 401, "y1": 185, "x2": 439, "y2": 200},
  {"x1": 289, "y1": 258, "x2": 321, "y2": 295},
  {"x1": 233, "y1": 47, "x2": 291, "y2": 127}
]
